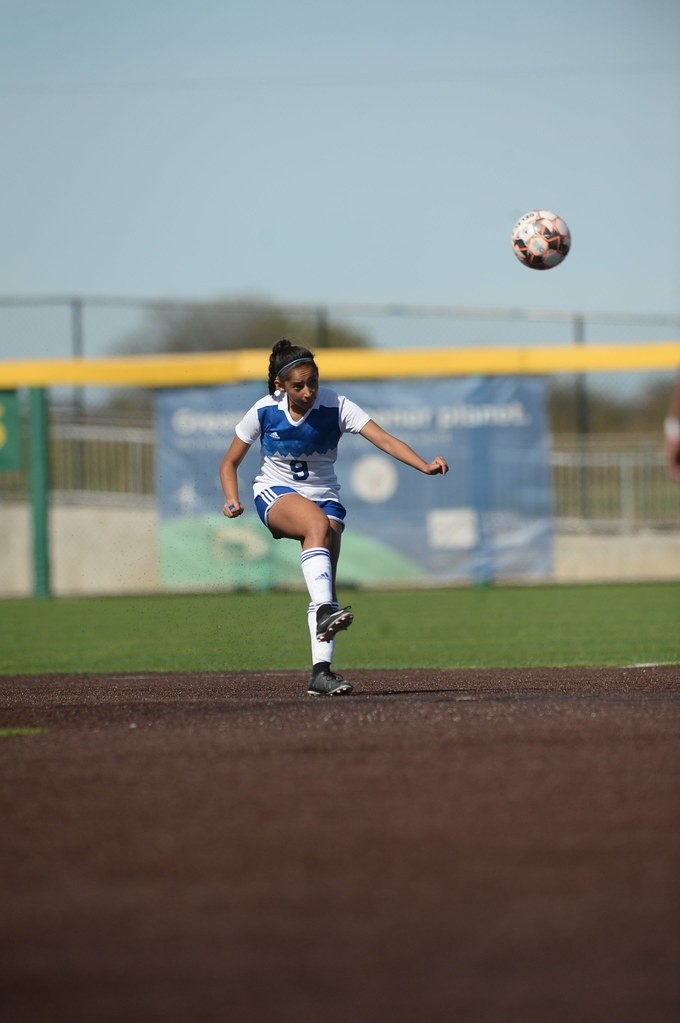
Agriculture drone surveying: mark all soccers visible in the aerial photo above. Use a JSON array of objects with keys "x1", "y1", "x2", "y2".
[{"x1": 509, "y1": 208, "x2": 572, "y2": 271}]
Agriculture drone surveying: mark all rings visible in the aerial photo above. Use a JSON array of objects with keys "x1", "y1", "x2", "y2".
[{"x1": 229, "y1": 505, "x2": 234, "y2": 510}]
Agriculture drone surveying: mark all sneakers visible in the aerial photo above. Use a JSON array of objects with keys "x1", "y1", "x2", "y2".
[
  {"x1": 308, "y1": 670, "x2": 353, "y2": 696},
  {"x1": 316, "y1": 606, "x2": 354, "y2": 642}
]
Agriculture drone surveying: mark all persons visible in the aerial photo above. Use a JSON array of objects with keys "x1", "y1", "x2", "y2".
[{"x1": 219, "y1": 338, "x2": 449, "y2": 696}]
